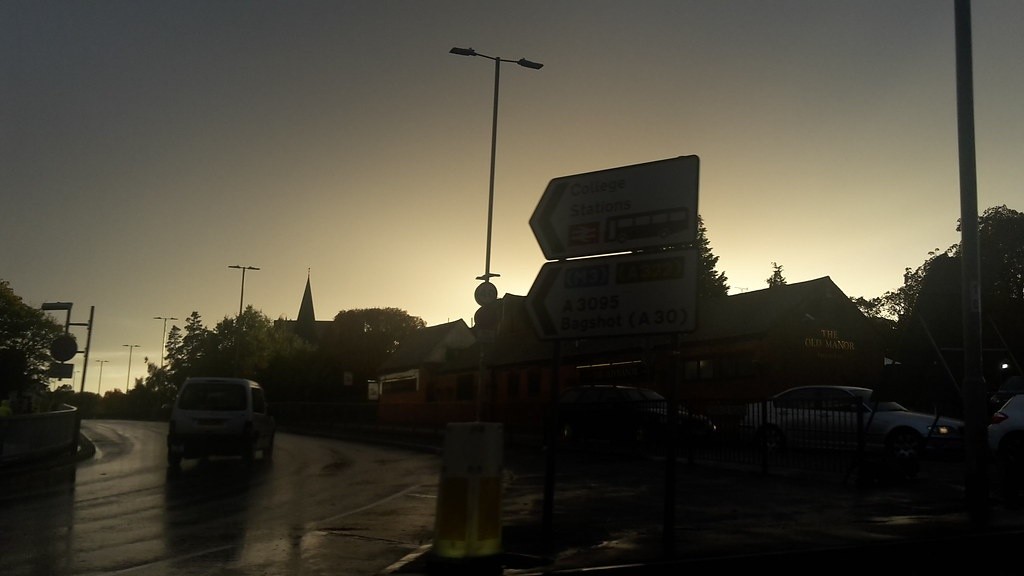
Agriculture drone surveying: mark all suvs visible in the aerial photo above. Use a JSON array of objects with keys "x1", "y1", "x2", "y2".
[{"x1": 167, "y1": 377, "x2": 276, "y2": 461}]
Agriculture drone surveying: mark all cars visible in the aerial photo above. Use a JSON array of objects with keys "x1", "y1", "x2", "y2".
[
  {"x1": 988, "y1": 391, "x2": 1024, "y2": 483},
  {"x1": 563, "y1": 384, "x2": 721, "y2": 459},
  {"x1": 737, "y1": 380, "x2": 967, "y2": 482}
]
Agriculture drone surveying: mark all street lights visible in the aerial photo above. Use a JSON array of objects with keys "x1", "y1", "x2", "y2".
[
  {"x1": 94, "y1": 315, "x2": 180, "y2": 397},
  {"x1": 228, "y1": 264, "x2": 261, "y2": 322},
  {"x1": 449, "y1": 46, "x2": 544, "y2": 322}
]
